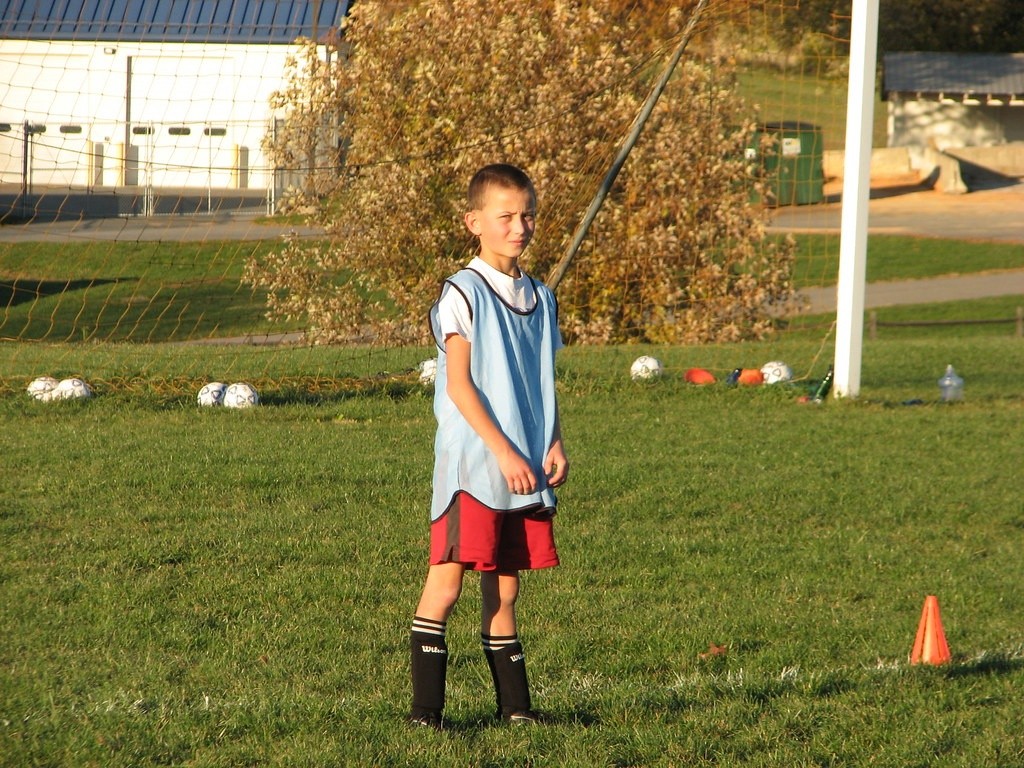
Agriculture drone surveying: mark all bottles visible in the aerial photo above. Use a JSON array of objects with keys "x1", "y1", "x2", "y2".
[
  {"x1": 812, "y1": 371, "x2": 834, "y2": 400},
  {"x1": 725, "y1": 367, "x2": 743, "y2": 386},
  {"x1": 939, "y1": 365, "x2": 963, "y2": 402}
]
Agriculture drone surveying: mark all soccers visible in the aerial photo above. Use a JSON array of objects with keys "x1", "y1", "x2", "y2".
[
  {"x1": 629, "y1": 355, "x2": 663, "y2": 389},
  {"x1": 418, "y1": 357, "x2": 438, "y2": 385},
  {"x1": 760, "y1": 360, "x2": 794, "y2": 386},
  {"x1": 26, "y1": 376, "x2": 91, "y2": 404},
  {"x1": 202, "y1": 382, "x2": 260, "y2": 411}
]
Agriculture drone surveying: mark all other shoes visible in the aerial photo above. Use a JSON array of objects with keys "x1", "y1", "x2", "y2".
[
  {"x1": 410, "y1": 716, "x2": 447, "y2": 732},
  {"x1": 495, "y1": 710, "x2": 539, "y2": 722}
]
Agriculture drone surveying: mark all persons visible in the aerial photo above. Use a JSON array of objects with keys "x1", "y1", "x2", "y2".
[{"x1": 409, "y1": 164, "x2": 568, "y2": 724}]
